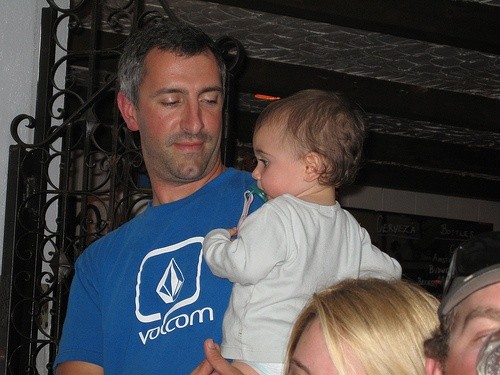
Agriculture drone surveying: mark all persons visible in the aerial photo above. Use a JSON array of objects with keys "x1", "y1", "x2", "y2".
[
  {"x1": 53, "y1": 18, "x2": 269, "y2": 375},
  {"x1": 283, "y1": 270, "x2": 440, "y2": 375},
  {"x1": 203, "y1": 89, "x2": 402, "y2": 375},
  {"x1": 424, "y1": 230, "x2": 500, "y2": 375}
]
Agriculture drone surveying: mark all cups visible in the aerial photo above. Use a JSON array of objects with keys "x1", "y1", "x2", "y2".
[{"x1": 476, "y1": 331, "x2": 500, "y2": 375}]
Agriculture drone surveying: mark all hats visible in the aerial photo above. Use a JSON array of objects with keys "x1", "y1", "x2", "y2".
[{"x1": 437, "y1": 231, "x2": 500, "y2": 318}]
